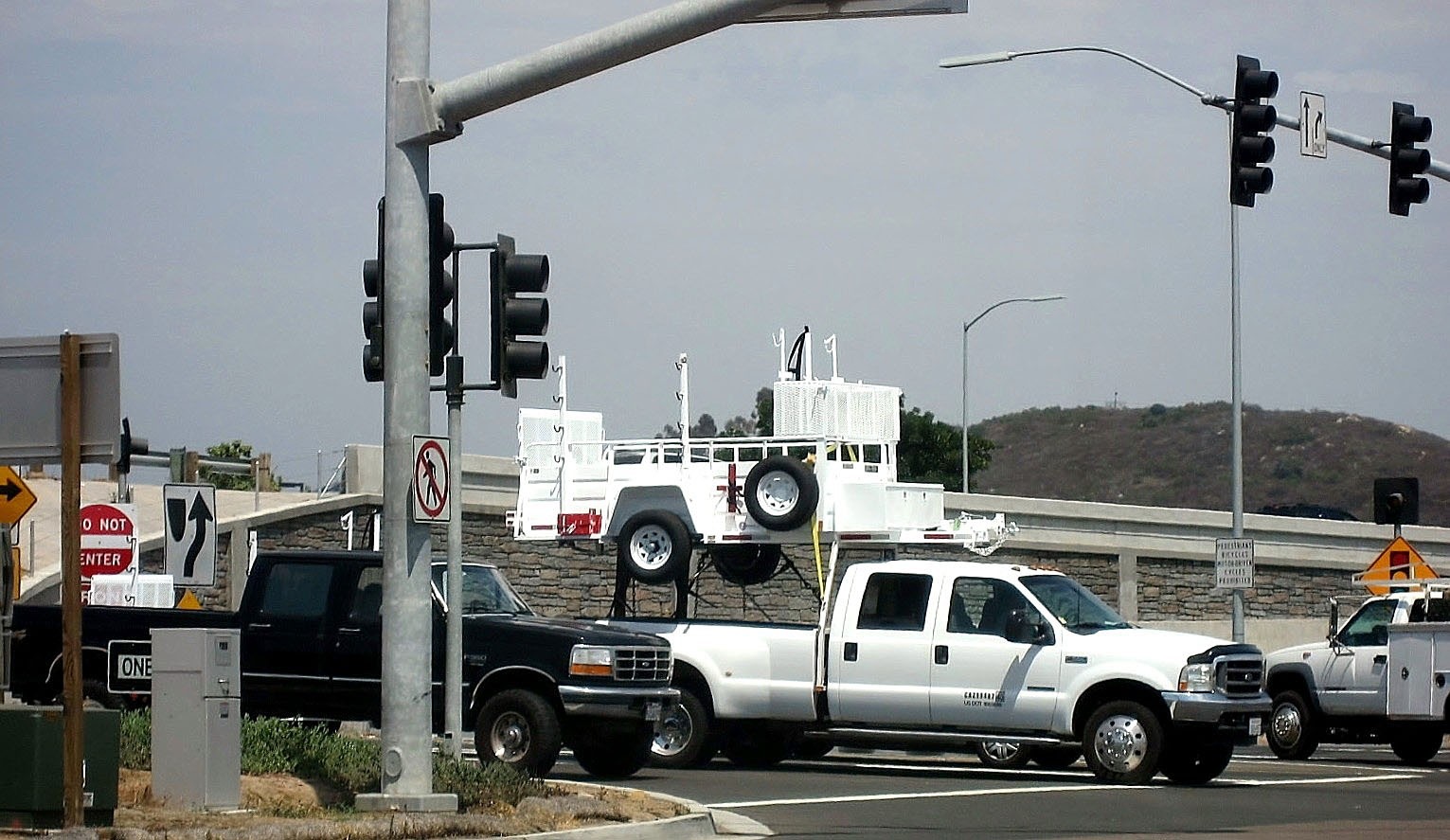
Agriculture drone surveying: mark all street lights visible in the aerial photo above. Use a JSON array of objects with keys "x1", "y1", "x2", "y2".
[
  {"x1": 963, "y1": 295, "x2": 1069, "y2": 492},
  {"x1": 939, "y1": 47, "x2": 1245, "y2": 647}
]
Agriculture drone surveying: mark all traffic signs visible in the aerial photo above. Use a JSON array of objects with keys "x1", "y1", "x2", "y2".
[{"x1": 1301, "y1": 91, "x2": 1328, "y2": 158}]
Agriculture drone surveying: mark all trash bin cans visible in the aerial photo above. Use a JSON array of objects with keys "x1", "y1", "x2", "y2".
[{"x1": 0, "y1": 704, "x2": 123, "y2": 827}]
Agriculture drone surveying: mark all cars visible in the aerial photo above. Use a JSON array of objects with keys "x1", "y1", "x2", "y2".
[{"x1": 1257, "y1": 503, "x2": 1359, "y2": 521}]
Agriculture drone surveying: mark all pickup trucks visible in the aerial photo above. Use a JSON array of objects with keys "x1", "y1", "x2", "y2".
[
  {"x1": 1244, "y1": 592, "x2": 1450, "y2": 764},
  {"x1": 10, "y1": 551, "x2": 673, "y2": 781},
  {"x1": 578, "y1": 559, "x2": 1273, "y2": 782}
]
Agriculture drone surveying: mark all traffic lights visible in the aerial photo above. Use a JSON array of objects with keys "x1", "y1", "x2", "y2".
[
  {"x1": 1231, "y1": 55, "x2": 1278, "y2": 207},
  {"x1": 490, "y1": 233, "x2": 549, "y2": 397},
  {"x1": 363, "y1": 192, "x2": 454, "y2": 381},
  {"x1": 1389, "y1": 102, "x2": 1432, "y2": 217}
]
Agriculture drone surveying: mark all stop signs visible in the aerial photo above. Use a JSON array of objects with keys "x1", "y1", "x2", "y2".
[{"x1": 80, "y1": 505, "x2": 134, "y2": 588}]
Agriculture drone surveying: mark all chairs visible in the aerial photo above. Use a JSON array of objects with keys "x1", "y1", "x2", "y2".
[
  {"x1": 978, "y1": 599, "x2": 1027, "y2": 637},
  {"x1": 946, "y1": 593, "x2": 977, "y2": 635},
  {"x1": 360, "y1": 582, "x2": 382, "y2": 618}
]
